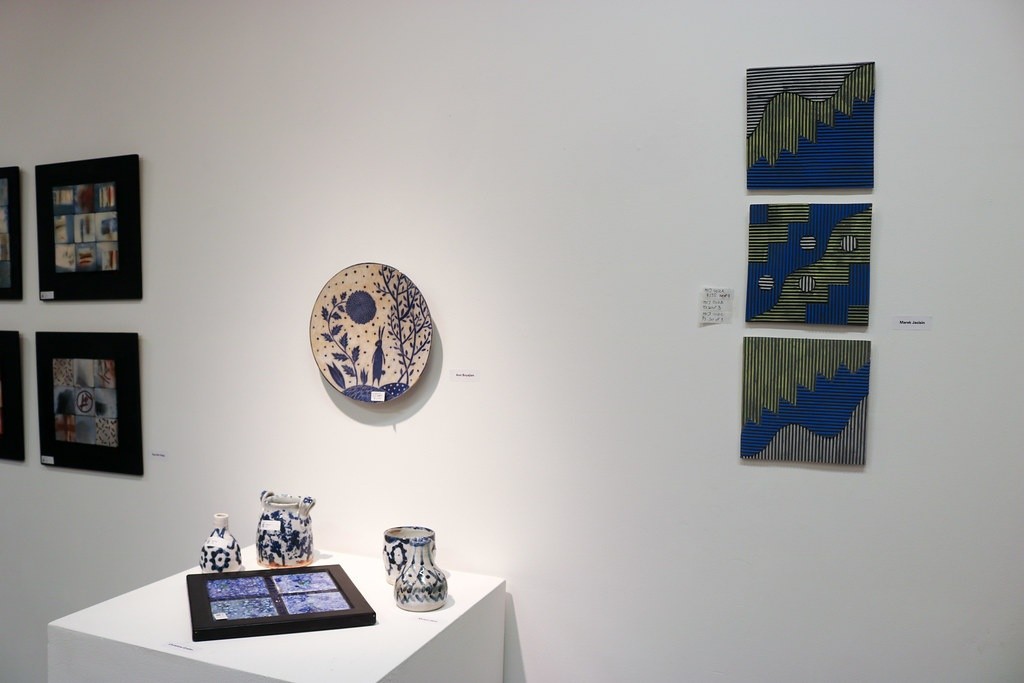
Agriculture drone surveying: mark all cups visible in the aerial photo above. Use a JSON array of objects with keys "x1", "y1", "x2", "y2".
[{"x1": 382, "y1": 525, "x2": 436, "y2": 585}]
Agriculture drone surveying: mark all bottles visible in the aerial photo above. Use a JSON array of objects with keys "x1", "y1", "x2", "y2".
[
  {"x1": 394, "y1": 536, "x2": 448, "y2": 611},
  {"x1": 199, "y1": 513, "x2": 242, "y2": 574}
]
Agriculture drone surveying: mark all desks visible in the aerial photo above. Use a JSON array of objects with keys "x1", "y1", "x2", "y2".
[{"x1": 45, "y1": 542, "x2": 509, "y2": 683}]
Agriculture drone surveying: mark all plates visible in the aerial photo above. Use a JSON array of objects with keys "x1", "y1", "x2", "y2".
[{"x1": 308, "y1": 262, "x2": 433, "y2": 404}]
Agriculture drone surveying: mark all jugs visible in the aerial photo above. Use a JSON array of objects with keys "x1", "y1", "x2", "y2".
[{"x1": 257, "y1": 491, "x2": 316, "y2": 569}]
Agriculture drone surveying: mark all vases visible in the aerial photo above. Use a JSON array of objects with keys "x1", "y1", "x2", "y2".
[
  {"x1": 255, "y1": 489, "x2": 316, "y2": 567},
  {"x1": 199, "y1": 513, "x2": 242, "y2": 573},
  {"x1": 384, "y1": 526, "x2": 438, "y2": 585},
  {"x1": 395, "y1": 537, "x2": 450, "y2": 612}
]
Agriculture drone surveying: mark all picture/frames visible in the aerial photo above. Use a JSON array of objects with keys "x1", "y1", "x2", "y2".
[
  {"x1": 34, "y1": 331, "x2": 144, "y2": 477},
  {"x1": 35, "y1": 153, "x2": 144, "y2": 302},
  {"x1": 0, "y1": 330, "x2": 26, "y2": 462},
  {"x1": 0, "y1": 165, "x2": 23, "y2": 302},
  {"x1": 186, "y1": 564, "x2": 376, "y2": 643}
]
